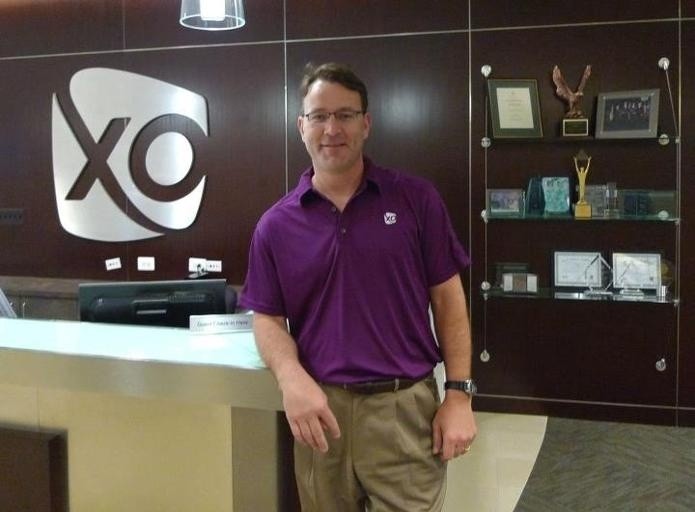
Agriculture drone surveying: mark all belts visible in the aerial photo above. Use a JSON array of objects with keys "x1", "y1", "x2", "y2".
[{"x1": 318, "y1": 368, "x2": 433, "y2": 395}]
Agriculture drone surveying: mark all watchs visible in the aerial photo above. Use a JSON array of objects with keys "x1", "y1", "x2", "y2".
[{"x1": 443, "y1": 378, "x2": 478, "y2": 397}]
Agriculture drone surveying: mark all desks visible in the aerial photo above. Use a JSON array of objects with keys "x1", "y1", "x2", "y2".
[{"x1": 0, "y1": 309, "x2": 294, "y2": 511}]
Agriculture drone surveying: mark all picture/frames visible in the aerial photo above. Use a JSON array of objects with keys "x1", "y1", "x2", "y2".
[
  {"x1": 487, "y1": 188, "x2": 525, "y2": 217},
  {"x1": 488, "y1": 79, "x2": 543, "y2": 138},
  {"x1": 595, "y1": 88, "x2": 660, "y2": 138}
]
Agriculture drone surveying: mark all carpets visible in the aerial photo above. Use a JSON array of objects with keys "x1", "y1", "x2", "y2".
[{"x1": 514, "y1": 417, "x2": 694, "y2": 512}]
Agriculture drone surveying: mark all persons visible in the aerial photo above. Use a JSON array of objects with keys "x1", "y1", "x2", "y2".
[
  {"x1": 605, "y1": 98, "x2": 649, "y2": 131},
  {"x1": 238, "y1": 62, "x2": 478, "y2": 511}
]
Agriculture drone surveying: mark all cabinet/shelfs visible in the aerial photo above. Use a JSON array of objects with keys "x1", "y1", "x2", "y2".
[{"x1": 482, "y1": 134, "x2": 679, "y2": 305}]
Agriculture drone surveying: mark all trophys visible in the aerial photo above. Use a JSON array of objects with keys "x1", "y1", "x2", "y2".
[
  {"x1": 552, "y1": 64, "x2": 592, "y2": 136},
  {"x1": 571, "y1": 155, "x2": 593, "y2": 217}
]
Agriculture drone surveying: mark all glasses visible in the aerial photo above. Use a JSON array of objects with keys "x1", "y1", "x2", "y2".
[{"x1": 304, "y1": 109, "x2": 363, "y2": 122}]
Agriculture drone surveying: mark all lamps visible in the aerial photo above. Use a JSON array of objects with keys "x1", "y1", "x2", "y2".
[{"x1": 179, "y1": 0, "x2": 246, "y2": 32}]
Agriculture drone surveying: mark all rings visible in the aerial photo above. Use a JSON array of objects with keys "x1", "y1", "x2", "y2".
[{"x1": 465, "y1": 446, "x2": 472, "y2": 452}]
